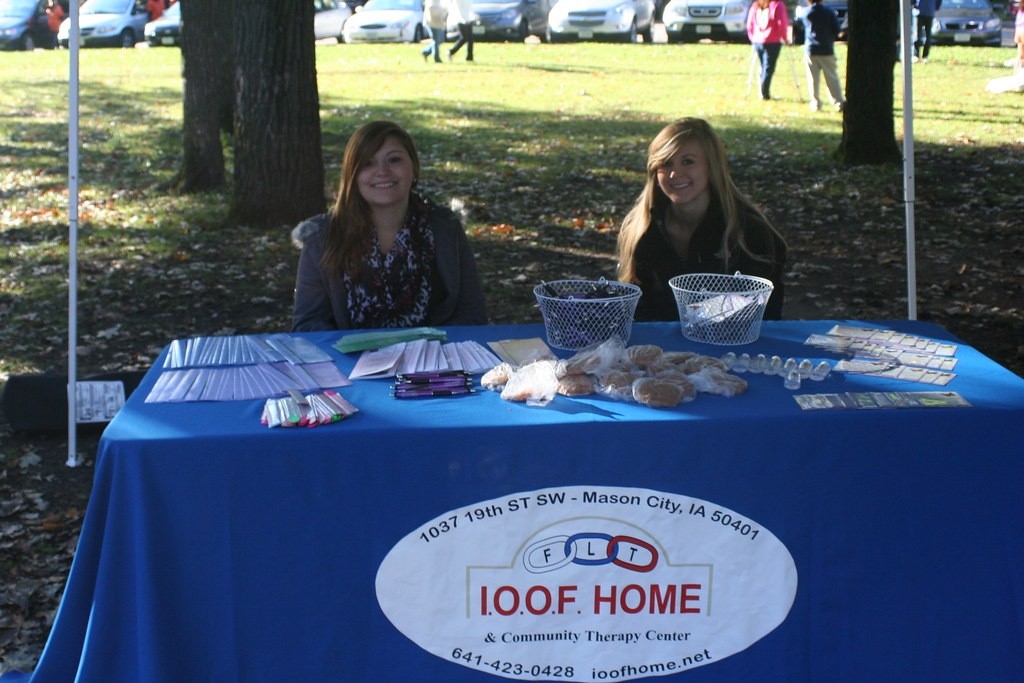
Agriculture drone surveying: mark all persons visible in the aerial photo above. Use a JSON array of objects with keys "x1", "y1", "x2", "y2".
[
  {"x1": 422, "y1": 0, "x2": 449, "y2": 62},
  {"x1": 144, "y1": 0, "x2": 166, "y2": 21},
  {"x1": 800, "y1": 0, "x2": 847, "y2": 113},
  {"x1": 291, "y1": 121, "x2": 489, "y2": 332},
  {"x1": 909, "y1": 0, "x2": 942, "y2": 63},
  {"x1": 615, "y1": 116, "x2": 788, "y2": 322},
  {"x1": 447, "y1": 0, "x2": 479, "y2": 62},
  {"x1": 746, "y1": 0, "x2": 789, "y2": 100},
  {"x1": 44, "y1": 0, "x2": 63, "y2": 48}
]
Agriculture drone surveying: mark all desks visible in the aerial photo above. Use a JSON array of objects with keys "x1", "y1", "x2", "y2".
[{"x1": 7, "y1": 319, "x2": 1024, "y2": 683}]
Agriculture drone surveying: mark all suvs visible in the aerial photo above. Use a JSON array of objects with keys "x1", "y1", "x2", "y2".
[{"x1": 0, "y1": 0, "x2": 70, "y2": 50}]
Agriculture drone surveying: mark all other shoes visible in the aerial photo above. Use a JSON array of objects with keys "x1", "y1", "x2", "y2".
[
  {"x1": 421, "y1": 50, "x2": 426, "y2": 62},
  {"x1": 912, "y1": 56, "x2": 929, "y2": 63},
  {"x1": 446, "y1": 49, "x2": 451, "y2": 63}
]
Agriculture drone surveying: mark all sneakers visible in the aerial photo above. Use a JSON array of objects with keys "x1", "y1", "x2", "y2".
[{"x1": 770, "y1": 96, "x2": 779, "y2": 101}]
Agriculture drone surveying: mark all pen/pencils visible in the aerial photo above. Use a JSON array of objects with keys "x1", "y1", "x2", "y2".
[
  {"x1": 389, "y1": 370, "x2": 477, "y2": 399},
  {"x1": 540, "y1": 278, "x2": 635, "y2": 348}
]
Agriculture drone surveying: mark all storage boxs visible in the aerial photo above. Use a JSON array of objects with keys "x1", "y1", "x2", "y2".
[{"x1": 6, "y1": 367, "x2": 148, "y2": 431}]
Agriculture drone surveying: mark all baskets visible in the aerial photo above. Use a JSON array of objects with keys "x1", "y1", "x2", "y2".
[
  {"x1": 669, "y1": 272, "x2": 774, "y2": 346},
  {"x1": 534, "y1": 276, "x2": 642, "y2": 352}
]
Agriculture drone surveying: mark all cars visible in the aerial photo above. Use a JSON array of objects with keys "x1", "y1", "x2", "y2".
[
  {"x1": 548, "y1": 0, "x2": 655, "y2": 43},
  {"x1": 344, "y1": 0, "x2": 428, "y2": 44},
  {"x1": 662, "y1": 0, "x2": 754, "y2": 43},
  {"x1": 57, "y1": 0, "x2": 150, "y2": 48},
  {"x1": 144, "y1": 0, "x2": 181, "y2": 47},
  {"x1": 314, "y1": 0, "x2": 366, "y2": 42},
  {"x1": 446, "y1": 0, "x2": 556, "y2": 43},
  {"x1": 913, "y1": 0, "x2": 1003, "y2": 47},
  {"x1": 792, "y1": 0, "x2": 849, "y2": 43}
]
